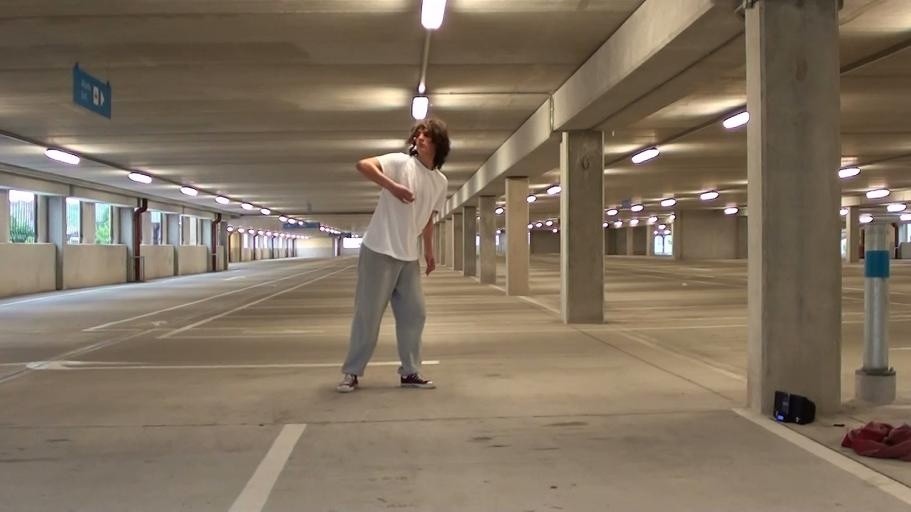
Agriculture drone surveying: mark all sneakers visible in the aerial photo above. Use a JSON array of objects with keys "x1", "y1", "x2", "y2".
[
  {"x1": 336, "y1": 373, "x2": 359, "y2": 393},
  {"x1": 400, "y1": 374, "x2": 436, "y2": 389}
]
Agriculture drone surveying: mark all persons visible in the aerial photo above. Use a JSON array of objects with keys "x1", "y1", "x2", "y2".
[{"x1": 336, "y1": 118, "x2": 450, "y2": 392}]
